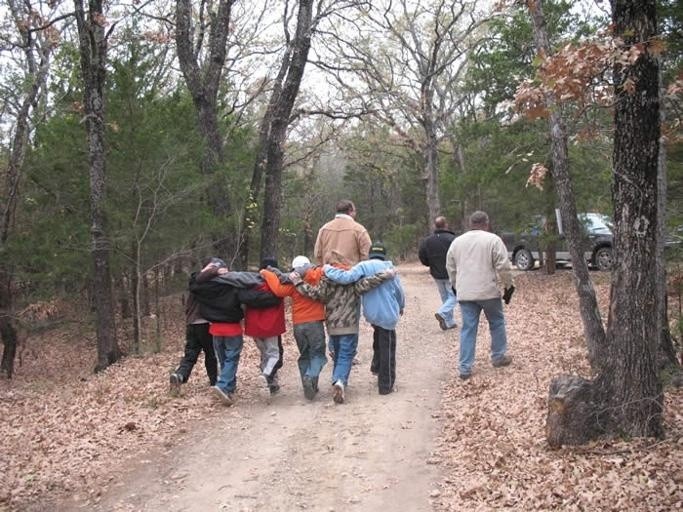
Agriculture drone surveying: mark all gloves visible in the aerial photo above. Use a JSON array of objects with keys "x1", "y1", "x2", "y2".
[{"x1": 503, "y1": 286, "x2": 514, "y2": 304}]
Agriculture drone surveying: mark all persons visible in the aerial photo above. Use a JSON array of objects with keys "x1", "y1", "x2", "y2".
[
  {"x1": 418, "y1": 216, "x2": 458, "y2": 330},
  {"x1": 314, "y1": 199, "x2": 372, "y2": 366},
  {"x1": 169, "y1": 242, "x2": 405, "y2": 406},
  {"x1": 445, "y1": 211, "x2": 515, "y2": 380}
]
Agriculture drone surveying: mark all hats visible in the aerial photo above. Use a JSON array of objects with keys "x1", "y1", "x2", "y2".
[
  {"x1": 369, "y1": 244, "x2": 386, "y2": 258},
  {"x1": 292, "y1": 256, "x2": 310, "y2": 268}
]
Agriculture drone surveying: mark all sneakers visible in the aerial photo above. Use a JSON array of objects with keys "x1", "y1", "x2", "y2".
[
  {"x1": 302, "y1": 378, "x2": 317, "y2": 399},
  {"x1": 493, "y1": 357, "x2": 512, "y2": 366},
  {"x1": 169, "y1": 374, "x2": 180, "y2": 395},
  {"x1": 459, "y1": 373, "x2": 470, "y2": 379},
  {"x1": 258, "y1": 373, "x2": 280, "y2": 403},
  {"x1": 436, "y1": 313, "x2": 457, "y2": 330},
  {"x1": 215, "y1": 387, "x2": 232, "y2": 405},
  {"x1": 333, "y1": 381, "x2": 344, "y2": 403}
]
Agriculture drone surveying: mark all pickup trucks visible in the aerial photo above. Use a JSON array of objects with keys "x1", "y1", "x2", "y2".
[{"x1": 501, "y1": 212, "x2": 615, "y2": 271}]
